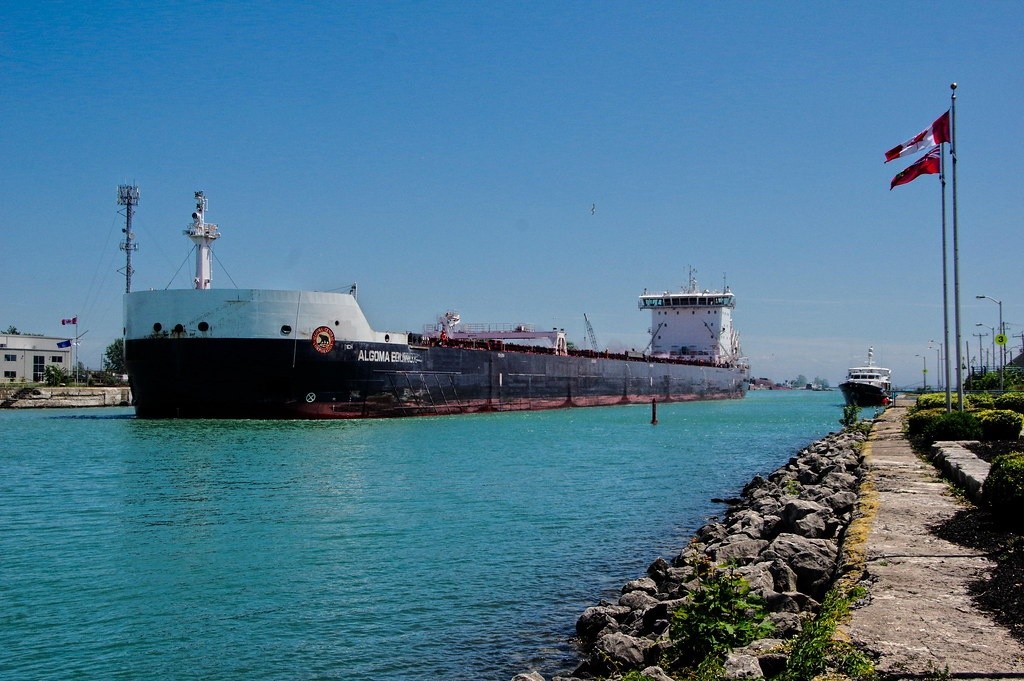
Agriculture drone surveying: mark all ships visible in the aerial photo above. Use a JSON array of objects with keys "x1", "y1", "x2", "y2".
[{"x1": 124, "y1": 188, "x2": 754, "y2": 421}]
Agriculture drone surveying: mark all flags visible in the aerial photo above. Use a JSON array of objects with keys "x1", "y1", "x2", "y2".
[
  {"x1": 890, "y1": 144, "x2": 940, "y2": 191},
  {"x1": 61, "y1": 317, "x2": 77, "y2": 325},
  {"x1": 884, "y1": 109, "x2": 950, "y2": 163},
  {"x1": 56, "y1": 340, "x2": 71, "y2": 348}
]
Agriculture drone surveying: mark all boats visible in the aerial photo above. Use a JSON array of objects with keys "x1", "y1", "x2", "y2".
[{"x1": 837, "y1": 345, "x2": 892, "y2": 406}]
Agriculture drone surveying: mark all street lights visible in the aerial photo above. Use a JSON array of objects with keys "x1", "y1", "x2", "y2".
[
  {"x1": 915, "y1": 354, "x2": 926, "y2": 393},
  {"x1": 931, "y1": 340, "x2": 943, "y2": 388},
  {"x1": 976, "y1": 323, "x2": 995, "y2": 371},
  {"x1": 976, "y1": 295, "x2": 1004, "y2": 389},
  {"x1": 929, "y1": 346, "x2": 939, "y2": 390}
]
